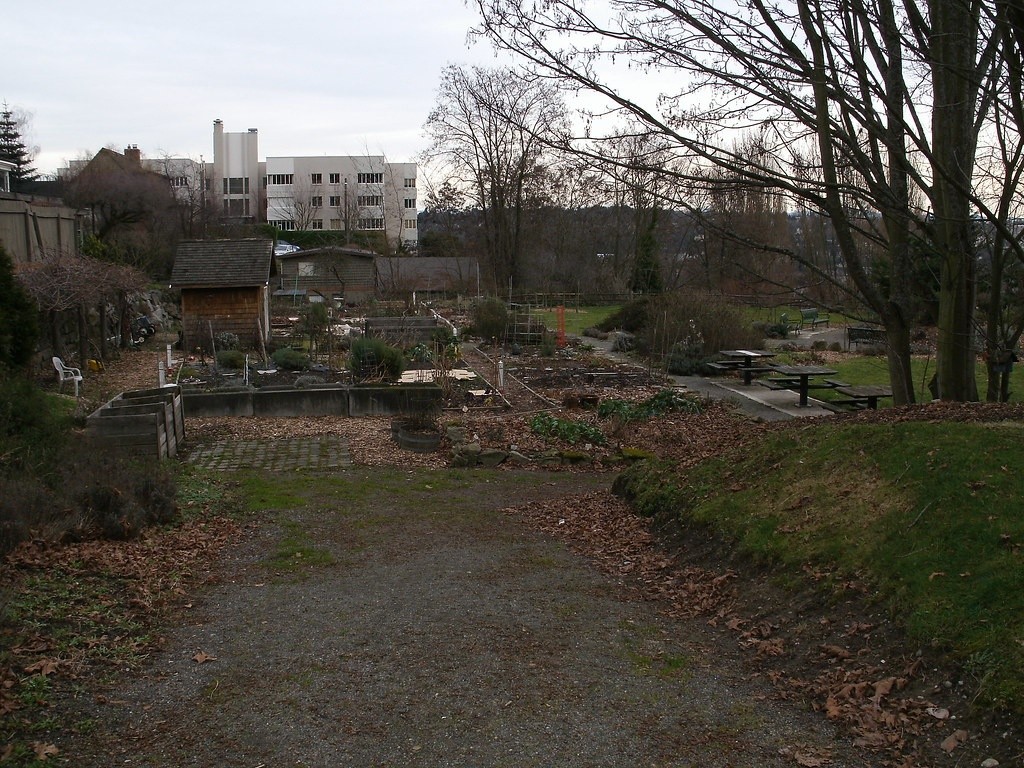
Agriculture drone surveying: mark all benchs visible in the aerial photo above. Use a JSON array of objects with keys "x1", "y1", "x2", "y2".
[
  {"x1": 828, "y1": 398, "x2": 882, "y2": 404},
  {"x1": 846, "y1": 328, "x2": 888, "y2": 351},
  {"x1": 778, "y1": 312, "x2": 801, "y2": 338},
  {"x1": 818, "y1": 401, "x2": 840, "y2": 414},
  {"x1": 758, "y1": 378, "x2": 853, "y2": 391},
  {"x1": 707, "y1": 360, "x2": 781, "y2": 372},
  {"x1": 800, "y1": 308, "x2": 831, "y2": 331}
]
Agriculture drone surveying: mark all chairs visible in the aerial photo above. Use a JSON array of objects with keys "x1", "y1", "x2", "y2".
[{"x1": 52, "y1": 357, "x2": 85, "y2": 397}]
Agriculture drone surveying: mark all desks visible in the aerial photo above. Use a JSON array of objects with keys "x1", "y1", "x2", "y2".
[
  {"x1": 773, "y1": 365, "x2": 838, "y2": 408},
  {"x1": 835, "y1": 385, "x2": 893, "y2": 411},
  {"x1": 719, "y1": 350, "x2": 778, "y2": 385}
]
